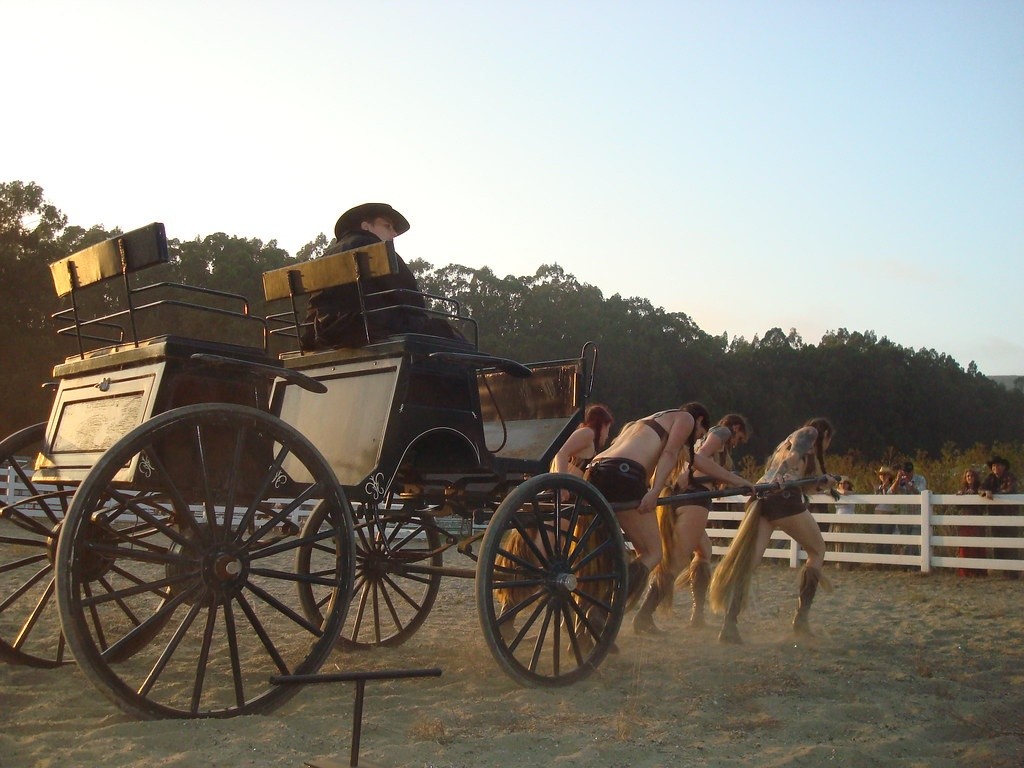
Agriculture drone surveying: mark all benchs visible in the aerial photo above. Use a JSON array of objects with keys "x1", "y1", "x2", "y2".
[
  {"x1": 263, "y1": 240, "x2": 491, "y2": 369},
  {"x1": 47, "y1": 222, "x2": 285, "y2": 377}
]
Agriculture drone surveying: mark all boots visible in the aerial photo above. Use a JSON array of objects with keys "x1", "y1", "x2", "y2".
[
  {"x1": 584, "y1": 565, "x2": 649, "y2": 655},
  {"x1": 687, "y1": 561, "x2": 711, "y2": 628},
  {"x1": 631, "y1": 569, "x2": 668, "y2": 635},
  {"x1": 496, "y1": 595, "x2": 538, "y2": 645},
  {"x1": 792, "y1": 566, "x2": 820, "y2": 640},
  {"x1": 717, "y1": 582, "x2": 750, "y2": 646},
  {"x1": 568, "y1": 584, "x2": 621, "y2": 658}
]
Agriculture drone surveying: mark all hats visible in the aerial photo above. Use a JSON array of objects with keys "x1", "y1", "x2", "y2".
[
  {"x1": 987, "y1": 455, "x2": 1010, "y2": 471},
  {"x1": 902, "y1": 461, "x2": 913, "y2": 472},
  {"x1": 874, "y1": 466, "x2": 896, "y2": 477},
  {"x1": 334, "y1": 203, "x2": 410, "y2": 240},
  {"x1": 835, "y1": 475, "x2": 854, "y2": 488}
]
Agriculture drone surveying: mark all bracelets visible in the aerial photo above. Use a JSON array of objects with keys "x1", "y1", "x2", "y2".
[{"x1": 985, "y1": 490, "x2": 992, "y2": 493}]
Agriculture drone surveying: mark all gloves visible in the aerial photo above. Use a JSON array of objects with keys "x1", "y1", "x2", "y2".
[{"x1": 830, "y1": 489, "x2": 841, "y2": 502}]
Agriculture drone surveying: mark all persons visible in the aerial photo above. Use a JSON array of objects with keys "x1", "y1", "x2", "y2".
[
  {"x1": 632, "y1": 410, "x2": 749, "y2": 635},
  {"x1": 954, "y1": 469, "x2": 986, "y2": 579},
  {"x1": 869, "y1": 466, "x2": 895, "y2": 572},
  {"x1": 302, "y1": 203, "x2": 455, "y2": 352},
  {"x1": 719, "y1": 469, "x2": 744, "y2": 514},
  {"x1": 718, "y1": 419, "x2": 835, "y2": 646},
  {"x1": 979, "y1": 456, "x2": 1022, "y2": 579},
  {"x1": 887, "y1": 462, "x2": 927, "y2": 573},
  {"x1": 568, "y1": 403, "x2": 756, "y2": 660},
  {"x1": 835, "y1": 476, "x2": 858, "y2": 570},
  {"x1": 501, "y1": 403, "x2": 612, "y2": 644}
]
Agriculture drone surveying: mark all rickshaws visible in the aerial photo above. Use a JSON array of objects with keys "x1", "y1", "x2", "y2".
[{"x1": 1, "y1": 221, "x2": 841, "y2": 723}]
